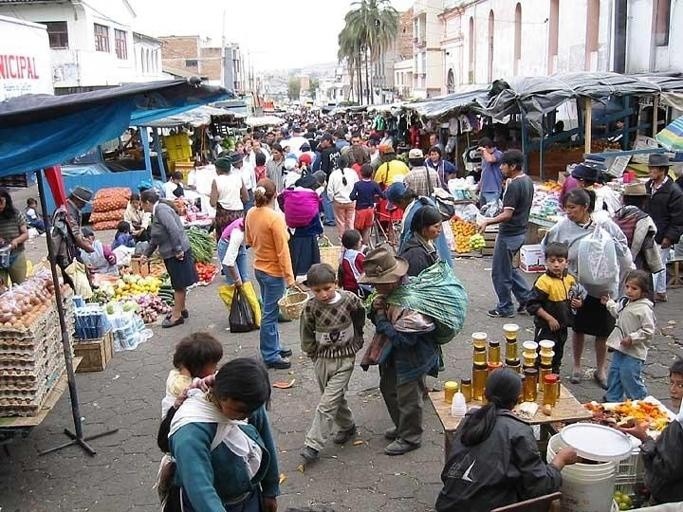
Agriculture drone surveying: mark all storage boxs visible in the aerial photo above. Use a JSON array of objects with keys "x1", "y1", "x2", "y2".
[
  {"x1": 518, "y1": 243, "x2": 547, "y2": 274},
  {"x1": 73, "y1": 327, "x2": 114, "y2": 373}
]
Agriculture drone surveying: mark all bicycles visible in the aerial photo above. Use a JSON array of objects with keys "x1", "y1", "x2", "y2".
[{"x1": 368, "y1": 203, "x2": 403, "y2": 254}]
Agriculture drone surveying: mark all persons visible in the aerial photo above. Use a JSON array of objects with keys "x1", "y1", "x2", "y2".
[
  {"x1": 0, "y1": 184, "x2": 200, "y2": 328},
  {"x1": 140, "y1": 104, "x2": 682, "y2": 512}
]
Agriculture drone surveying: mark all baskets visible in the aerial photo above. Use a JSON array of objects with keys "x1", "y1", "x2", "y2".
[
  {"x1": 316, "y1": 234, "x2": 342, "y2": 273},
  {"x1": 167, "y1": 145, "x2": 192, "y2": 160},
  {"x1": 164, "y1": 133, "x2": 190, "y2": 149},
  {"x1": 278, "y1": 286, "x2": 310, "y2": 320},
  {"x1": 616, "y1": 453, "x2": 639, "y2": 477},
  {"x1": 166, "y1": 157, "x2": 190, "y2": 173},
  {"x1": 615, "y1": 479, "x2": 638, "y2": 497}
]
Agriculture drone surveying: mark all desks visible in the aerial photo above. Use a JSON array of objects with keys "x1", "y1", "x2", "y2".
[
  {"x1": 1, "y1": 355, "x2": 85, "y2": 454},
  {"x1": 427, "y1": 380, "x2": 597, "y2": 467}
]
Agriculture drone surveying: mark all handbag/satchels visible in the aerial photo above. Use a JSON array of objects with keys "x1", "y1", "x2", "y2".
[
  {"x1": 218, "y1": 281, "x2": 261, "y2": 329},
  {"x1": 430, "y1": 193, "x2": 455, "y2": 222}
]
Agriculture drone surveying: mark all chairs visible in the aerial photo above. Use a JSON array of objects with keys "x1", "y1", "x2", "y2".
[{"x1": 486, "y1": 492, "x2": 566, "y2": 511}]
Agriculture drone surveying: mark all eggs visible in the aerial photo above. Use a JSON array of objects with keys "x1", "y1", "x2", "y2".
[{"x1": 0, "y1": 269, "x2": 75, "y2": 417}]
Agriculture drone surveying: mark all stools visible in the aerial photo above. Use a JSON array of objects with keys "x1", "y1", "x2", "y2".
[{"x1": 665, "y1": 256, "x2": 683, "y2": 290}]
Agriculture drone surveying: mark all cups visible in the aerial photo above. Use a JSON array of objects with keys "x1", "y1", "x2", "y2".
[
  {"x1": 538, "y1": 339, "x2": 554, "y2": 354},
  {"x1": 471, "y1": 332, "x2": 486, "y2": 348},
  {"x1": 539, "y1": 350, "x2": 555, "y2": 364},
  {"x1": 502, "y1": 323, "x2": 519, "y2": 339},
  {"x1": 522, "y1": 341, "x2": 538, "y2": 356},
  {"x1": 522, "y1": 352, "x2": 538, "y2": 366}
]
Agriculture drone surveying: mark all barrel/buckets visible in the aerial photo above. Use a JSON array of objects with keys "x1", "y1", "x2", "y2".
[{"x1": 544, "y1": 421, "x2": 634, "y2": 512}]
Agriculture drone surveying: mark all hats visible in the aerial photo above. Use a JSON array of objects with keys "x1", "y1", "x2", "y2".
[
  {"x1": 295, "y1": 175, "x2": 317, "y2": 188},
  {"x1": 646, "y1": 154, "x2": 674, "y2": 167},
  {"x1": 373, "y1": 143, "x2": 386, "y2": 153},
  {"x1": 408, "y1": 149, "x2": 424, "y2": 159},
  {"x1": 71, "y1": 185, "x2": 93, "y2": 205},
  {"x1": 356, "y1": 247, "x2": 409, "y2": 285},
  {"x1": 385, "y1": 182, "x2": 413, "y2": 210},
  {"x1": 571, "y1": 162, "x2": 598, "y2": 182},
  {"x1": 317, "y1": 133, "x2": 332, "y2": 140},
  {"x1": 231, "y1": 153, "x2": 243, "y2": 163},
  {"x1": 383, "y1": 145, "x2": 395, "y2": 154}
]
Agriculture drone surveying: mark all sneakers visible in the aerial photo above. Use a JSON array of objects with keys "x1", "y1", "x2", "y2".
[
  {"x1": 488, "y1": 310, "x2": 515, "y2": 318},
  {"x1": 517, "y1": 301, "x2": 532, "y2": 314},
  {"x1": 384, "y1": 437, "x2": 421, "y2": 456},
  {"x1": 384, "y1": 425, "x2": 400, "y2": 438},
  {"x1": 334, "y1": 424, "x2": 356, "y2": 444},
  {"x1": 299, "y1": 446, "x2": 319, "y2": 462}
]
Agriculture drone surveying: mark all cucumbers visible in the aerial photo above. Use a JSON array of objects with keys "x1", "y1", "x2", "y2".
[{"x1": 157, "y1": 285, "x2": 175, "y2": 306}]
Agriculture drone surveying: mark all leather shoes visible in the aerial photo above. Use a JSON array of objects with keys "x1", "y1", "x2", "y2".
[
  {"x1": 570, "y1": 368, "x2": 582, "y2": 384},
  {"x1": 166, "y1": 308, "x2": 188, "y2": 319},
  {"x1": 162, "y1": 316, "x2": 184, "y2": 328},
  {"x1": 594, "y1": 370, "x2": 609, "y2": 391},
  {"x1": 280, "y1": 348, "x2": 292, "y2": 358},
  {"x1": 265, "y1": 358, "x2": 291, "y2": 369}
]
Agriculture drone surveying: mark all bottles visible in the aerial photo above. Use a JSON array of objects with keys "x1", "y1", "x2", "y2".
[
  {"x1": 459, "y1": 376, "x2": 471, "y2": 402},
  {"x1": 444, "y1": 381, "x2": 458, "y2": 403},
  {"x1": 522, "y1": 368, "x2": 538, "y2": 402},
  {"x1": 504, "y1": 336, "x2": 517, "y2": 358},
  {"x1": 472, "y1": 345, "x2": 487, "y2": 365},
  {"x1": 542, "y1": 374, "x2": 557, "y2": 407},
  {"x1": 503, "y1": 358, "x2": 522, "y2": 404},
  {"x1": 538, "y1": 362, "x2": 552, "y2": 392},
  {"x1": 471, "y1": 362, "x2": 488, "y2": 401},
  {"x1": 487, "y1": 361, "x2": 502, "y2": 373},
  {"x1": 487, "y1": 341, "x2": 500, "y2": 365},
  {"x1": 552, "y1": 374, "x2": 560, "y2": 399}
]
